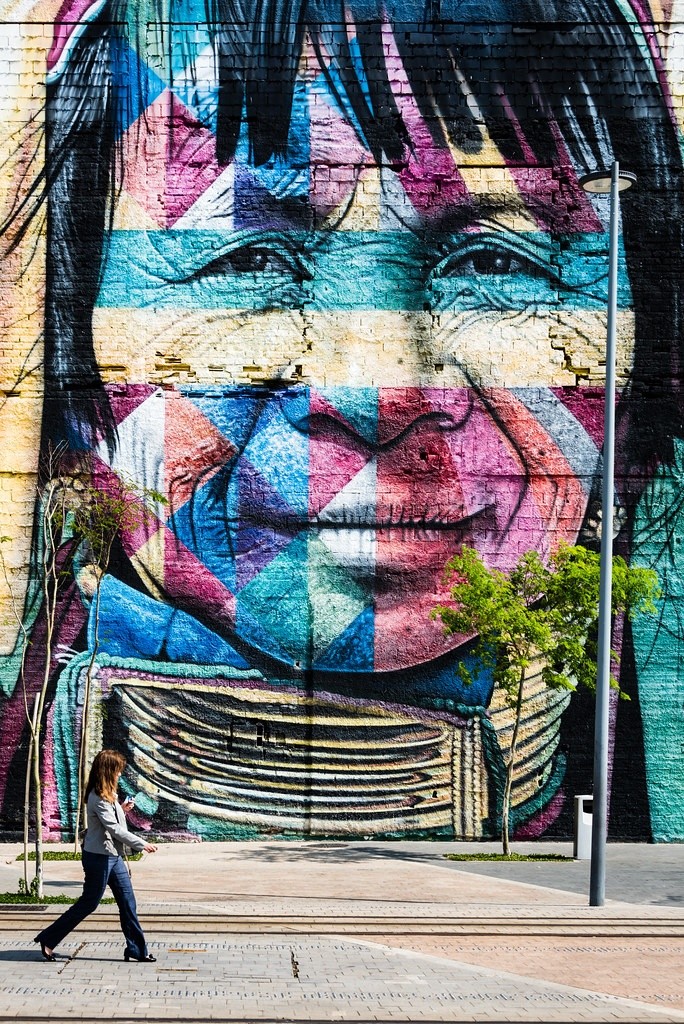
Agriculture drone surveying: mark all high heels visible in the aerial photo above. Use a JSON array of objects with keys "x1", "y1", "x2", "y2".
[
  {"x1": 124, "y1": 952, "x2": 156, "y2": 963},
  {"x1": 34, "y1": 934, "x2": 57, "y2": 961}
]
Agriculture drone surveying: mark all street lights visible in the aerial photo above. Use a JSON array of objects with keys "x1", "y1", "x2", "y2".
[{"x1": 578, "y1": 160, "x2": 639, "y2": 906}]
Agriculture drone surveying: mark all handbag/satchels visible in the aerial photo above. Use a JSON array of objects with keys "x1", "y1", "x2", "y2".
[{"x1": 121, "y1": 845, "x2": 144, "y2": 862}]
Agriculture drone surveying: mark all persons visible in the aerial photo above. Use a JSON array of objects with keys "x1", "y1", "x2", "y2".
[{"x1": 33, "y1": 749, "x2": 158, "y2": 963}]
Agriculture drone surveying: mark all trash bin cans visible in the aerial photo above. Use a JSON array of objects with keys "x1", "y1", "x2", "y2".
[{"x1": 573, "y1": 794, "x2": 595, "y2": 860}]
